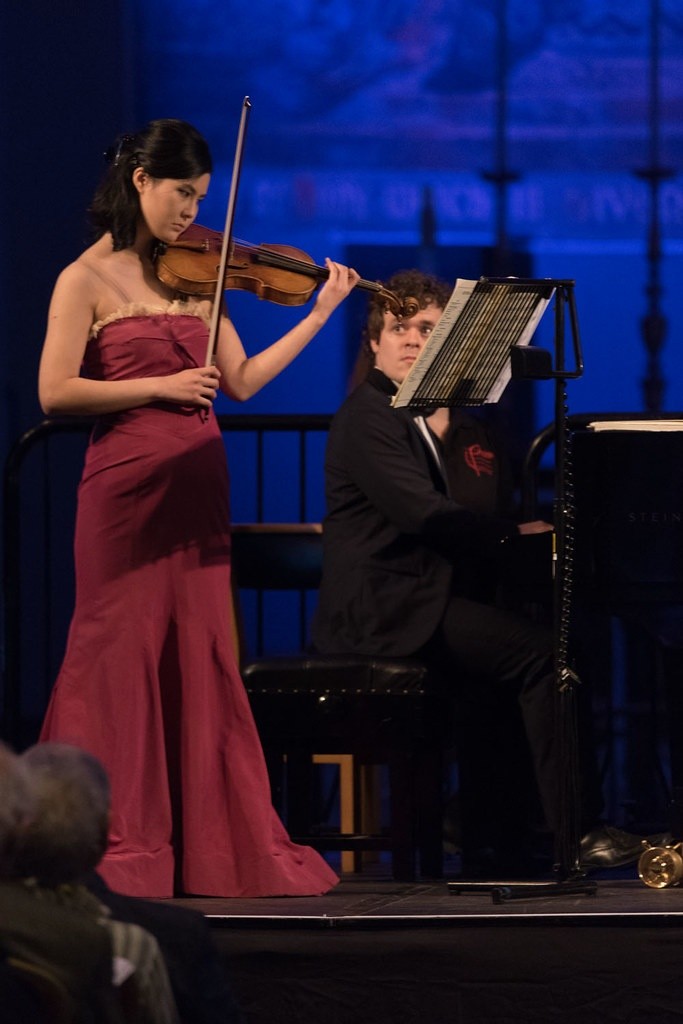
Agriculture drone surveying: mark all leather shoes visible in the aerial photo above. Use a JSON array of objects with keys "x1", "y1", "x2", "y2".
[{"x1": 550, "y1": 825, "x2": 674, "y2": 878}]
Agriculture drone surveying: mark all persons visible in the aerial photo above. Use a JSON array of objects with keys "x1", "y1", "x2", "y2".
[
  {"x1": 39, "y1": 119, "x2": 362, "y2": 899},
  {"x1": 308, "y1": 271, "x2": 673, "y2": 882},
  {"x1": 0, "y1": 741, "x2": 221, "y2": 1024}
]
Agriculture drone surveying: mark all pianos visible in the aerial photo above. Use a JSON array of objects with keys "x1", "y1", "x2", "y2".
[{"x1": 527, "y1": 412, "x2": 683, "y2": 891}]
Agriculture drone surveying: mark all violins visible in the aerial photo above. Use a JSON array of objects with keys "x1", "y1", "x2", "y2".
[{"x1": 157, "y1": 222, "x2": 420, "y2": 328}]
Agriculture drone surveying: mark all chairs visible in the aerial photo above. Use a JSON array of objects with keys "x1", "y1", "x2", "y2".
[{"x1": 227, "y1": 523, "x2": 450, "y2": 888}]
